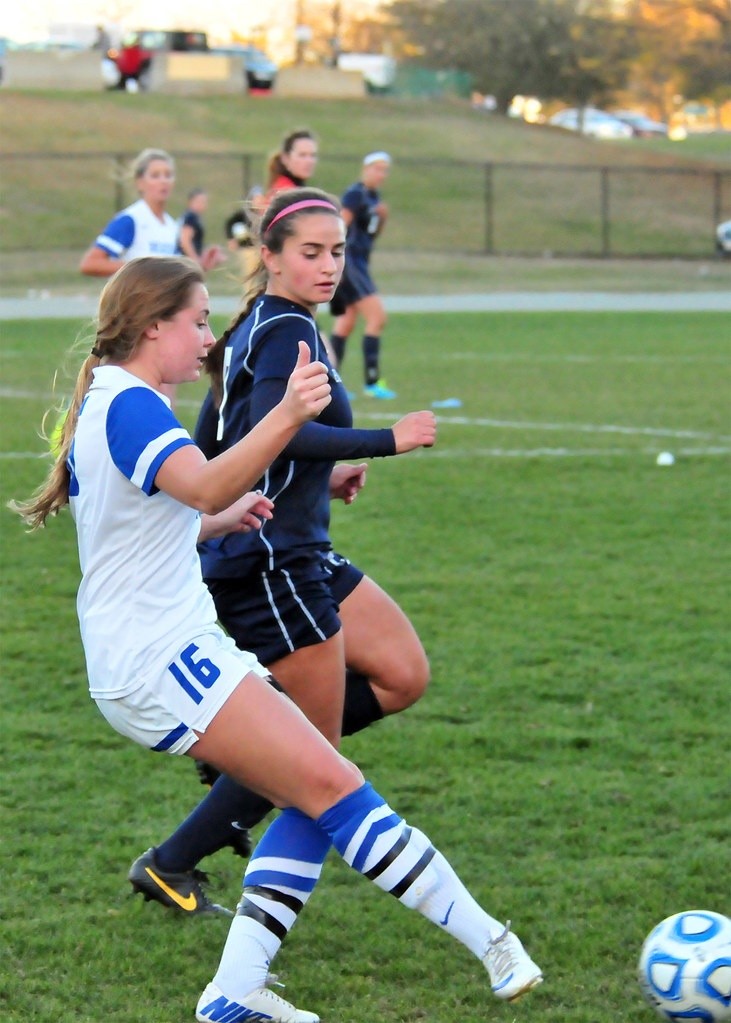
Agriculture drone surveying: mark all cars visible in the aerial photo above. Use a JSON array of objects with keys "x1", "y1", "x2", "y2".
[{"x1": 203, "y1": 36, "x2": 675, "y2": 149}]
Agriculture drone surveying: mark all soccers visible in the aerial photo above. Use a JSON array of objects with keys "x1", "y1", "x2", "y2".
[{"x1": 641, "y1": 909, "x2": 731, "y2": 1023}]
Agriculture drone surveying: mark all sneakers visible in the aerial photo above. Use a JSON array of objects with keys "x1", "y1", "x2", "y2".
[
  {"x1": 347, "y1": 391, "x2": 356, "y2": 400},
  {"x1": 480, "y1": 920, "x2": 543, "y2": 1002},
  {"x1": 194, "y1": 982, "x2": 319, "y2": 1023},
  {"x1": 365, "y1": 381, "x2": 396, "y2": 400},
  {"x1": 196, "y1": 760, "x2": 252, "y2": 858},
  {"x1": 128, "y1": 845, "x2": 235, "y2": 920}
]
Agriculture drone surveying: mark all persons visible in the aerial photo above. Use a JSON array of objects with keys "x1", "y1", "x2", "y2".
[
  {"x1": 85, "y1": 145, "x2": 224, "y2": 281},
  {"x1": 9, "y1": 258, "x2": 544, "y2": 1023},
  {"x1": 330, "y1": 148, "x2": 401, "y2": 402},
  {"x1": 256, "y1": 125, "x2": 340, "y2": 378},
  {"x1": 180, "y1": 181, "x2": 210, "y2": 254},
  {"x1": 227, "y1": 185, "x2": 267, "y2": 299},
  {"x1": 125, "y1": 185, "x2": 434, "y2": 920}
]
